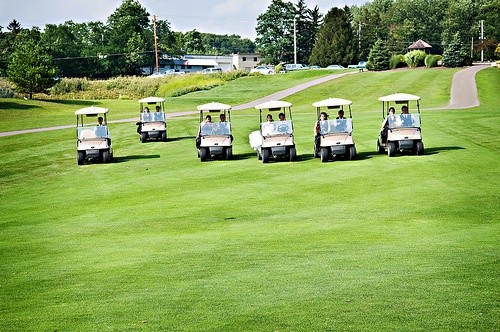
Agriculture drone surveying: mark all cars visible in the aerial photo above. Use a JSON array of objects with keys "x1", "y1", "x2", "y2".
[
  {"x1": 249, "y1": 66, "x2": 274, "y2": 75},
  {"x1": 146, "y1": 72, "x2": 166, "y2": 78},
  {"x1": 309, "y1": 66, "x2": 321, "y2": 70},
  {"x1": 327, "y1": 65, "x2": 344, "y2": 69}
]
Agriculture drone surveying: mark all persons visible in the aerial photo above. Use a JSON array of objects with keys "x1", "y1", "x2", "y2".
[
  {"x1": 381, "y1": 106, "x2": 398, "y2": 131},
  {"x1": 313, "y1": 112, "x2": 333, "y2": 157},
  {"x1": 275, "y1": 113, "x2": 291, "y2": 132},
  {"x1": 196, "y1": 115, "x2": 217, "y2": 138},
  {"x1": 333, "y1": 110, "x2": 351, "y2": 131},
  {"x1": 153, "y1": 105, "x2": 166, "y2": 121},
  {"x1": 95, "y1": 117, "x2": 109, "y2": 138},
  {"x1": 396, "y1": 106, "x2": 416, "y2": 127},
  {"x1": 142, "y1": 107, "x2": 154, "y2": 122},
  {"x1": 217, "y1": 114, "x2": 233, "y2": 143},
  {"x1": 261, "y1": 113, "x2": 277, "y2": 134}
]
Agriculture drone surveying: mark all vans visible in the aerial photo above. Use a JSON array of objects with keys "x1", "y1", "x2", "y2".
[
  {"x1": 202, "y1": 68, "x2": 222, "y2": 74},
  {"x1": 286, "y1": 63, "x2": 307, "y2": 69}
]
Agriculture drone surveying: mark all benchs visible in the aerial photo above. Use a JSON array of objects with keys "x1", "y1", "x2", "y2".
[{"x1": 80, "y1": 130, "x2": 96, "y2": 137}]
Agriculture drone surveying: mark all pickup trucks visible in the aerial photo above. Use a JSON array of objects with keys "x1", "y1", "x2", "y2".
[
  {"x1": 348, "y1": 61, "x2": 367, "y2": 69},
  {"x1": 164, "y1": 69, "x2": 190, "y2": 75}
]
